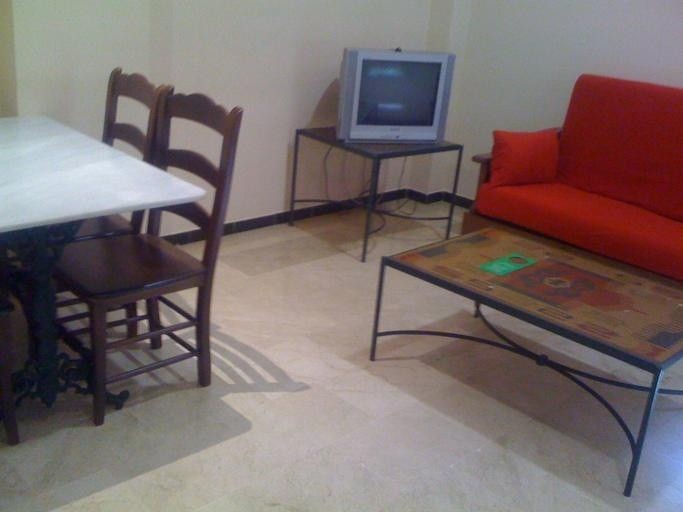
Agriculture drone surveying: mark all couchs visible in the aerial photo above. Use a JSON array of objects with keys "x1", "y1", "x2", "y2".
[{"x1": 453, "y1": 73, "x2": 683, "y2": 289}]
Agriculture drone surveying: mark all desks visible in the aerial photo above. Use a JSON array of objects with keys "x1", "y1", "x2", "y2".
[
  {"x1": 286, "y1": 124, "x2": 463, "y2": 267},
  {"x1": 0, "y1": 112, "x2": 210, "y2": 446}
]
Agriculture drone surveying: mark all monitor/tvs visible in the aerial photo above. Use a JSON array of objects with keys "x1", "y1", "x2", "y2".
[{"x1": 335, "y1": 46, "x2": 456, "y2": 147}]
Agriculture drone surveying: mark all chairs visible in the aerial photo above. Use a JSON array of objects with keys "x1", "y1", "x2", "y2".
[
  {"x1": 24, "y1": 83, "x2": 245, "y2": 429},
  {"x1": 0, "y1": 64, "x2": 171, "y2": 362}
]
